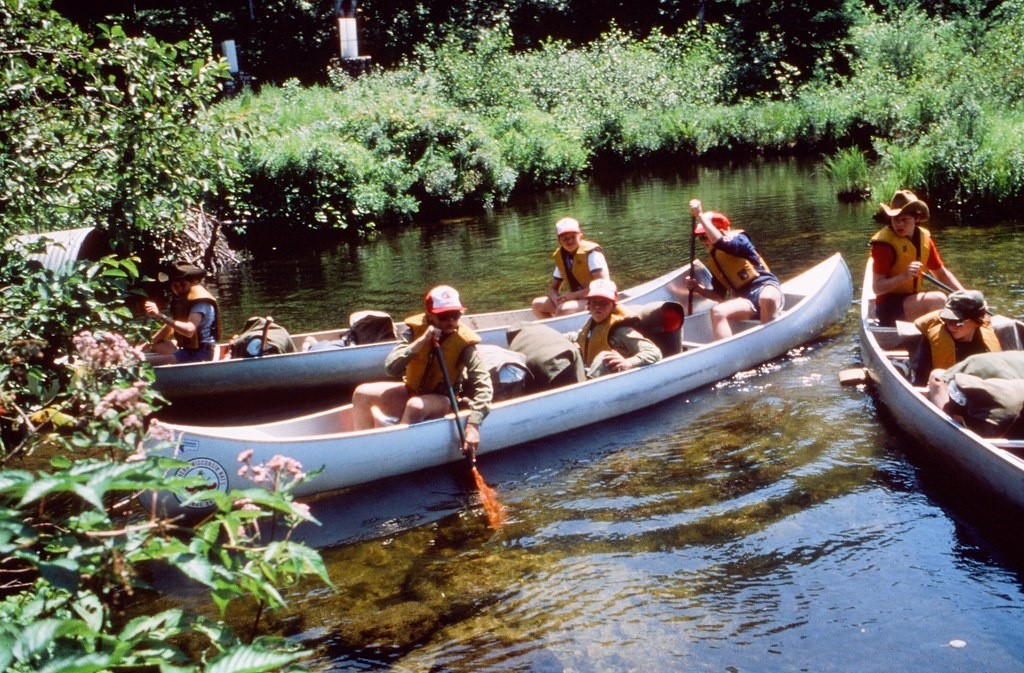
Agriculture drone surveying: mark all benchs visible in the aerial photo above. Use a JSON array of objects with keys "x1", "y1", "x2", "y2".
[
  {"x1": 872, "y1": 322, "x2": 1024, "y2": 446},
  {"x1": 683, "y1": 319, "x2": 760, "y2": 349}
]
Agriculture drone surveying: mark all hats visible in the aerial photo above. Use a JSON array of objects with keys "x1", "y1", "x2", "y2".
[
  {"x1": 939, "y1": 289, "x2": 988, "y2": 321},
  {"x1": 873, "y1": 190, "x2": 930, "y2": 224},
  {"x1": 157, "y1": 260, "x2": 202, "y2": 282},
  {"x1": 556, "y1": 217, "x2": 580, "y2": 236},
  {"x1": 425, "y1": 285, "x2": 468, "y2": 313},
  {"x1": 695, "y1": 211, "x2": 729, "y2": 234},
  {"x1": 585, "y1": 278, "x2": 618, "y2": 302}
]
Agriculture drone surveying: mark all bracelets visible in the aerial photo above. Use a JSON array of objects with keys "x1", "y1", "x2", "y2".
[{"x1": 160, "y1": 315, "x2": 166, "y2": 323}]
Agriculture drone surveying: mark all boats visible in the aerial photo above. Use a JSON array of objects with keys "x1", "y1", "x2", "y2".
[
  {"x1": 128, "y1": 252, "x2": 854, "y2": 524},
  {"x1": 54, "y1": 259, "x2": 713, "y2": 408},
  {"x1": 839, "y1": 254, "x2": 1024, "y2": 505}
]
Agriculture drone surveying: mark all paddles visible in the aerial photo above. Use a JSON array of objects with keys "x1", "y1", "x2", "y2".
[
  {"x1": 685, "y1": 197, "x2": 698, "y2": 316},
  {"x1": 257, "y1": 315, "x2": 274, "y2": 358},
  {"x1": 912, "y1": 265, "x2": 996, "y2": 317},
  {"x1": 430, "y1": 330, "x2": 507, "y2": 533},
  {"x1": 131, "y1": 308, "x2": 154, "y2": 349}
]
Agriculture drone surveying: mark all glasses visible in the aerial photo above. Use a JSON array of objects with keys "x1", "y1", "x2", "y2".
[
  {"x1": 947, "y1": 319, "x2": 966, "y2": 327},
  {"x1": 439, "y1": 312, "x2": 458, "y2": 322}
]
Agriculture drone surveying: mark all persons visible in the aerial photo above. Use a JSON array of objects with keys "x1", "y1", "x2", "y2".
[
  {"x1": 566, "y1": 278, "x2": 663, "y2": 379},
  {"x1": 869, "y1": 190, "x2": 989, "y2": 328},
  {"x1": 687, "y1": 198, "x2": 784, "y2": 341},
  {"x1": 353, "y1": 285, "x2": 494, "y2": 462},
  {"x1": 911, "y1": 289, "x2": 1002, "y2": 418},
  {"x1": 532, "y1": 217, "x2": 610, "y2": 319},
  {"x1": 145, "y1": 260, "x2": 218, "y2": 366}
]
